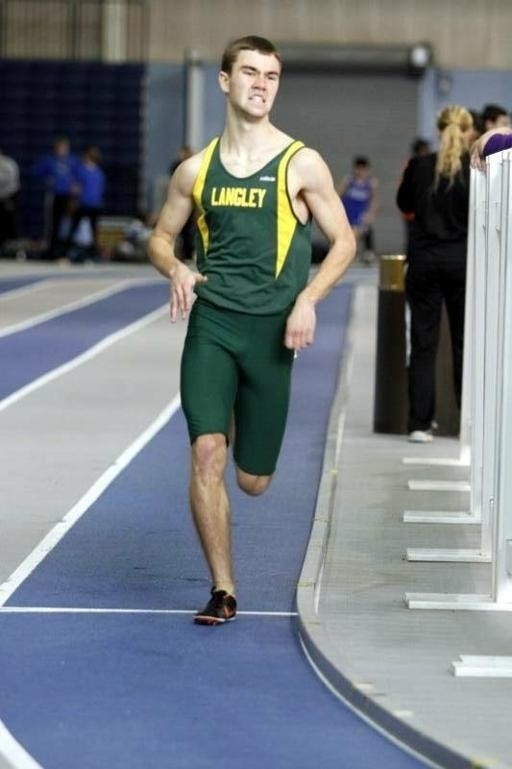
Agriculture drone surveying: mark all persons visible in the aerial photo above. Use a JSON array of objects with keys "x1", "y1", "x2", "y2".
[
  {"x1": 395, "y1": 104, "x2": 478, "y2": 443},
  {"x1": 336, "y1": 154, "x2": 381, "y2": 252},
  {"x1": 168, "y1": 144, "x2": 203, "y2": 260},
  {"x1": 468, "y1": 104, "x2": 512, "y2": 173},
  {"x1": 144, "y1": 32, "x2": 359, "y2": 627},
  {"x1": 409, "y1": 133, "x2": 434, "y2": 156},
  {"x1": 33, "y1": 135, "x2": 109, "y2": 262}
]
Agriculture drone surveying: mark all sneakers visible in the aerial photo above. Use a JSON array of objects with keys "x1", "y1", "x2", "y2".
[{"x1": 192, "y1": 586, "x2": 237, "y2": 626}]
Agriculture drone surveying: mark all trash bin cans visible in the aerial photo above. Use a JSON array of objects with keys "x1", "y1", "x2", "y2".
[{"x1": 374, "y1": 256, "x2": 460, "y2": 437}]
